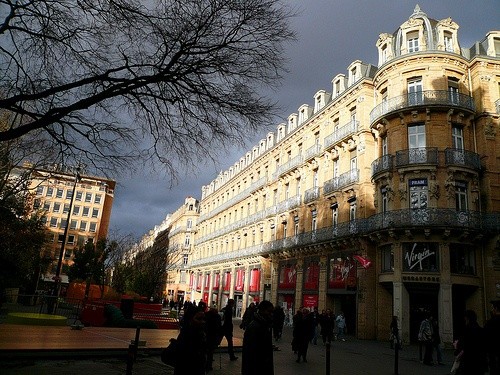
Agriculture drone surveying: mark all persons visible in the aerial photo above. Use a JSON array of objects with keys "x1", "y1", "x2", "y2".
[
  {"x1": 390, "y1": 316, "x2": 402, "y2": 350},
  {"x1": 46, "y1": 285, "x2": 56, "y2": 313},
  {"x1": 416, "y1": 301, "x2": 500, "y2": 375},
  {"x1": 166, "y1": 298, "x2": 347, "y2": 375}
]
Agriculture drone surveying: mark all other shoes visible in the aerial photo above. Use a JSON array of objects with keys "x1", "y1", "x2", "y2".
[
  {"x1": 303, "y1": 360, "x2": 307, "y2": 363},
  {"x1": 295, "y1": 359, "x2": 301, "y2": 363},
  {"x1": 335, "y1": 336, "x2": 337, "y2": 341},
  {"x1": 341, "y1": 338, "x2": 345, "y2": 342},
  {"x1": 294, "y1": 351, "x2": 297, "y2": 355},
  {"x1": 230, "y1": 357, "x2": 238, "y2": 361}
]
